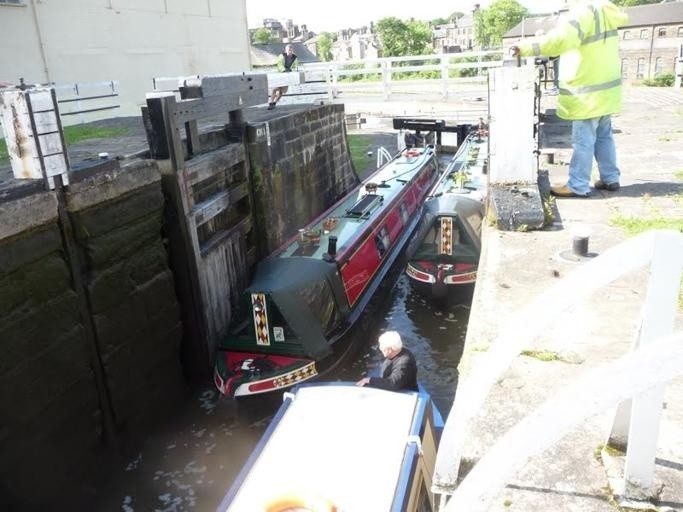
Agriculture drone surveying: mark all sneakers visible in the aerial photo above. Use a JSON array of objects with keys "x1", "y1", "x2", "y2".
[
  {"x1": 550, "y1": 186, "x2": 590, "y2": 197},
  {"x1": 595, "y1": 180, "x2": 620, "y2": 191}
]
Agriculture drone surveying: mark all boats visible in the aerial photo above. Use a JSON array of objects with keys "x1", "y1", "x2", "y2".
[
  {"x1": 404, "y1": 130, "x2": 489, "y2": 300},
  {"x1": 214, "y1": 366, "x2": 445, "y2": 512},
  {"x1": 211, "y1": 145, "x2": 442, "y2": 400}
]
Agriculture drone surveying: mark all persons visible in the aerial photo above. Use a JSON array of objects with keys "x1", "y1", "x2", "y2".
[
  {"x1": 354, "y1": 330, "x2": 418, "y2": 391},
  {"x1": 266, "y1": 44, "x2": 302, "y2": 110},
  {"x1": 476, "y1": 117, "x2": 486, "y2": 137},
  {"x1": 509, "y1": 0, "x2": 631, "y2": 198},
  {"x1": 435, "y1": 263, "x2": 446, "y2": 286}
]
